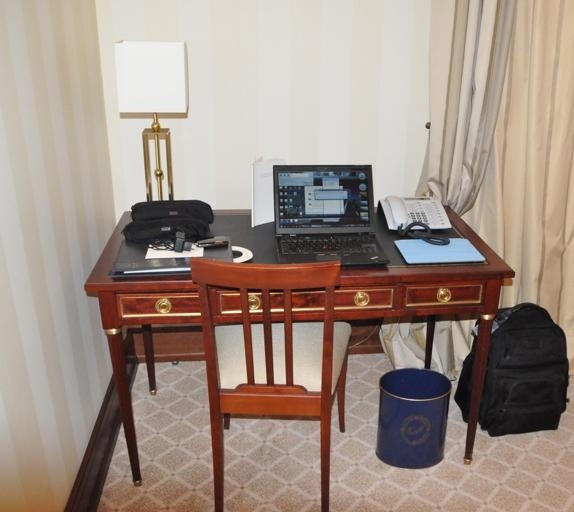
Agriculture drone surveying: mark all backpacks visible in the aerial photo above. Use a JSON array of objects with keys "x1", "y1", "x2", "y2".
[{"x1": 454, "y1": 303, "x2": 570, "y2": 438}]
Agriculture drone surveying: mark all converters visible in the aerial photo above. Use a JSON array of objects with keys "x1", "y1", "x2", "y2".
[{"x1": 174, "y1": 231, "x2": 192, "y2": 252}]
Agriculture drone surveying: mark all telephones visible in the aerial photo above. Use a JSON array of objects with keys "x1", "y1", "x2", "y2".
[{"x1": 377, "y1": 195, "x2": 453, "y2": 232}]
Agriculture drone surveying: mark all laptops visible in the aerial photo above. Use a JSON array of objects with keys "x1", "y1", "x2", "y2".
[{"x1": 273, "y1": 165, "x2": 390, "y2": 267}]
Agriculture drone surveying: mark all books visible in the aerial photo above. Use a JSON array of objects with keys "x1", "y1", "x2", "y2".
[{"x1": 251, "y1": 157, "x2": 286, "y2": 228}]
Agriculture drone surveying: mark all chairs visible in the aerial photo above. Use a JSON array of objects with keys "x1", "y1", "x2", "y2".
[{"x1": 189, "y1": 257, "x2": 353, "y2": 512}]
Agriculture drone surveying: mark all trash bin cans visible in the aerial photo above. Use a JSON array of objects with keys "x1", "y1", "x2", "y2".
[{"x1": 375, "y1": 368, "x2": 451, "y2": 469}]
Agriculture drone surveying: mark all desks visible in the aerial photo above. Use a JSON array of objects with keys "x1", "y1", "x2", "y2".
[{"x1": 84, "y1": 205, "x2": 516, "y2": 488}]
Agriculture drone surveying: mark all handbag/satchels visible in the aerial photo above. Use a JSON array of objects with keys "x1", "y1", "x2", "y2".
[{"x1": 122, "y1": 200, "x2": 214, "y2": 252}]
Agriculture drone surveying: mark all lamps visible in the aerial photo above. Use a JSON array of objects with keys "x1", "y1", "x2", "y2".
[{"x1": 114, "y1": 38, "x2": 190, "y2": 202}]
